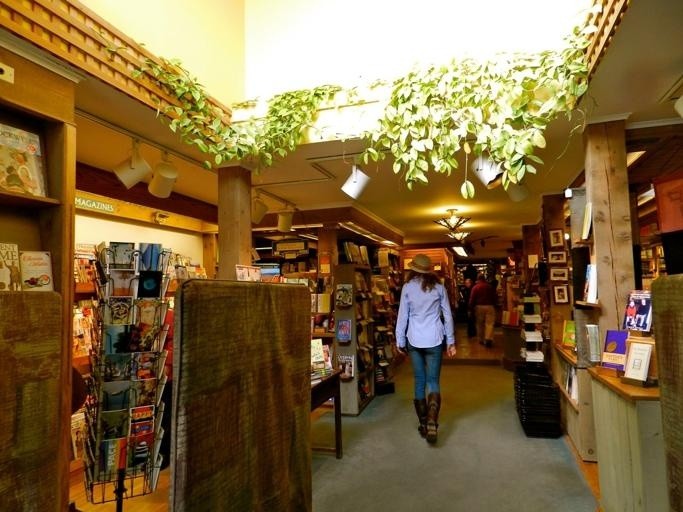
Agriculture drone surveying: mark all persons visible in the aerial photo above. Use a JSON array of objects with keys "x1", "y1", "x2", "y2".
[
  {"x1": 396, "y1": 254, "x2": 457, "y2": 445},
  {"x1": 468, "y1": 273, "x2": 498, "y2": 348},
  {"x1": 634, "y1": 299, "x2": 648, "y2": 328},
  {"x1": 626, "y1": 301, "x2": 637, "y2": 326},
  {"x1": 463, "y1": 278, "x2": 477, "y2": 337}
]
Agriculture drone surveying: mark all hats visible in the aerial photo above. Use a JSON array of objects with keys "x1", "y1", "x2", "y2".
[{"x1": 408, "y1": 254, "x2": 433, "y2": 274}]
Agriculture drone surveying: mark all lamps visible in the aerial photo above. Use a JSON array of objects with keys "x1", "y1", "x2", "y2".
[
  {"x1": 433, "y1": 209, "x2": 472, "y2": 231},
  {"x1": 565, "y1": 185, "x2": 573, "y2": 198},
  {"x1": 443, "y1": 232, "x2": 473, "y2": 242},
  {"x1": 471, "y1": 150, "x2": 505, "y2": 190}
]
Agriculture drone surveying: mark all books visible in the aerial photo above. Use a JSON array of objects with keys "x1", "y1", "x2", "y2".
[
  {"x1": 0, "y1": 243, "x2": 21, "y2": 293},
  {"x1": 521, "y1": 314, "x2": 542, "y2": 324},
  {"x1": 0, "y1": 122, "x2": 48, "y2": 198},
  {"x1": 521, "y1": 329, "x2": 543, "y2": 342},
  {"x1": 337, "y1": 320, "x2": 351, "y2": 341},
  {"x1": 19, "y1": 251, "x2": 54, "y2": 292},
  {"x1": 336, "y1": 354, "x2": 354, "y2": 379},
  {"x1": 80, "y1": 243, "x2": 170, "y2": 495},
  {"x1": 336, "y1": 284, "x2": 353, "y2": 306},
  {"x1": 623, "y1": 343, "x2": 652, "y2": 381},
  {"x1": 519, "y1": 347, "x2": 544, "y2": 363},
  {"x1": 518, "y1": 296, "x2": 541, "y2": 303},
  {"x1": 602, "y1": 330, "x2": 630, "y2": 372}
]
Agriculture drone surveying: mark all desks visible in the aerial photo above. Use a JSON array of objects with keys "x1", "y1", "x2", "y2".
[{"x1": 587, "y1": 366, "x2": 671, "y2": 512}]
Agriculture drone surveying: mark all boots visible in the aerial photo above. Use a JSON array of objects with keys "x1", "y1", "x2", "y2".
[
  {"x1": 414, "y1": 399, "x2": 427, "y2": 438},
  {"x1": 426, "y1": 393, "x2": 441, "y2": 442}
]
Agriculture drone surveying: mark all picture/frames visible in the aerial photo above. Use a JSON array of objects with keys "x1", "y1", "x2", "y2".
[
  {"x1": 547, "y1": 228, "x2": 570, "y2": 305},
  {"x1": 622, "y1": 290, "x2": 653, "y2": 333}
]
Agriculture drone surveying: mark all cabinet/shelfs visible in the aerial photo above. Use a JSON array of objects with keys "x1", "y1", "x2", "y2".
[
  {"x1": 575, "y1": 239, "x2": 599, "y2": 308},
  {"x1": 554, "y1": 344, "x2": 596, "y2": 463},
  {"x1": 506, "y1": 282, "x2": 524, "y2": 311},
  {"x1": 519, "y1": 291, "x2": 544, "y2": 366}
]
